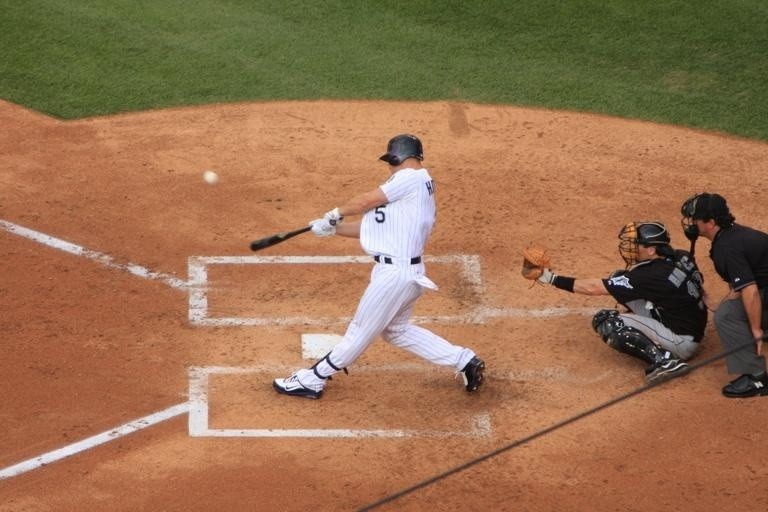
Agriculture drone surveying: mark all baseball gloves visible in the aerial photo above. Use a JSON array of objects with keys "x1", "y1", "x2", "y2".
[{"x1": 521, "y1": 243, "x2": 551, "y2": 278}]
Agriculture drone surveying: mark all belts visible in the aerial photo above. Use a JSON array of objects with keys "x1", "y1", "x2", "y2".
[{"x1": 375, "y1": 256, "x2": 421, "y2": 264}]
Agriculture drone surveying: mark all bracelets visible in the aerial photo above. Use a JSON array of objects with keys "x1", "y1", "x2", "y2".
[{"x1": 555, "y1": 276, "x2": 577, "y2": 293}]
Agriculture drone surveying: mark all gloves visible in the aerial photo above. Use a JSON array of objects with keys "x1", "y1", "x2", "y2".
[
  {"x1": 309, "y1": 208, "x2": 344, "y2": 237},
  {"x1": 536, "y1": 268, "x2": 557, "y2": 285}
]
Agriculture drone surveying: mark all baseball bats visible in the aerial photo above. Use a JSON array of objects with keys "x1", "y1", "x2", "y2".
[{"x1": 250, "y1": 218, "x2": 337, "y2": 252}]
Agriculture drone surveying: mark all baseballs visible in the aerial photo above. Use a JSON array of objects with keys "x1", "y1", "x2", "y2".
[{"x1": 204, "y1": 169, "x2": 218, "y2": 185}]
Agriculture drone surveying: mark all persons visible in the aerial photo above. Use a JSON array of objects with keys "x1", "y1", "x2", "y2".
[
  {"x1": 271, "y1": 134, "x2": 484, "y2": 400},
  {"x1": 522, "y1": 220, "x2": 707, "y2": 385},
  {"x1": 680, "y1": 192, "x2": 768, "y2": 398}
]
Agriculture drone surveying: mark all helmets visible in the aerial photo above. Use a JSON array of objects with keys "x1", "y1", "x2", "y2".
[
  {"x1": 619, "y1": 222, "x2": 671, "y2": 270},
  {"x1": 681, "y1": 193, "x2": 726, "y2": 257},
  {"x1": 379, "y1": 134, "x2": 423, "y2": 166}
]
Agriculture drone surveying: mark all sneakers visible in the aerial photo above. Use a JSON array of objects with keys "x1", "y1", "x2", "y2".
[
  {"x1": 722, "y1": 371, "x2": 768, "y2": 398},
  {"x1": 273, "y1": 375, "x2": 322, "y2": 399},
  {"x1": 455, "y1": 356, "x2": 485, "y2": 391},
  {"x1": 645, "y1": 359, "x2": 688, "y2": 384}
]
